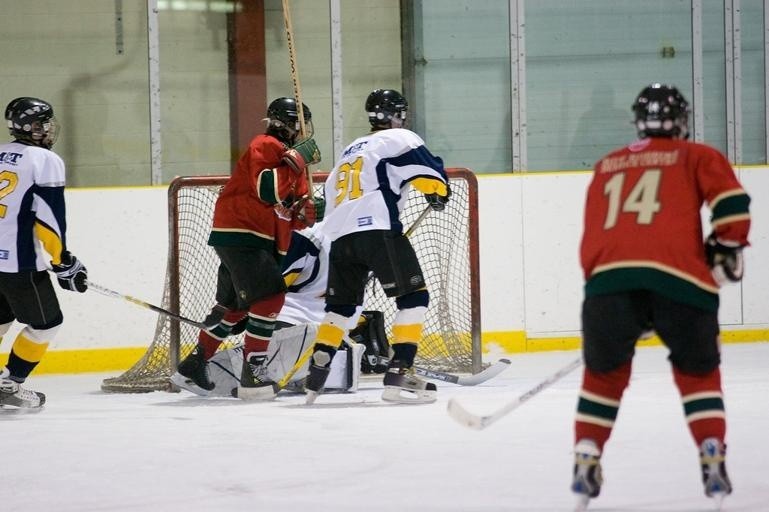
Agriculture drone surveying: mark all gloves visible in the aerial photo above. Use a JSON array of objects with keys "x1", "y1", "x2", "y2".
[
  {"x1": 425, "y1": 185, "x2": 452, "y2": 211},
  {"x1": 51, "y1": 256, "x2": 89, "y2": 293},
  {"x1": 283, "y1": 138, "x2": 322, "y2": 173},
  {"x1": 295, "y1": 196, "x2": 327, "y2": 223}
]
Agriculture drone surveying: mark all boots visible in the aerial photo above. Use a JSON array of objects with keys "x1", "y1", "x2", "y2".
[
  {"x1": 700, "y1": 438, "x2": 733, "y2": 496},
  {"x1": 383, "y1": 348, "x2": 436, "y2": 391},
  {"x1": 572, "y1": 440, "x2": 604, "y2": 498},
  {"x1": 306, "y1": 351, "x2": 331, "y2": 390},
  {"x1": 241, "y1": 349, "x2": 279, "y2": 387},
  {"x1": 1, "y1": 367, "x2": 46, "y2": 409},
  {"x1": 177, "y1": 344, "x2": 215, "y2": 390}
]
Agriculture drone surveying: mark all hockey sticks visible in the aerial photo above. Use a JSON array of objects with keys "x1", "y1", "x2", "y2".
[
  {"x1": 47, "y1": 265, "x2": 225, "y2": 331},
  {"x1": 363, "y1": 358, "x2": 511, "y2": 386},
  {"x1": 447, "y1": 355, "x2": 582, "y2": 432},
  {"x1": 231, "y1": 204, "x2": 429, "y2": 400}
]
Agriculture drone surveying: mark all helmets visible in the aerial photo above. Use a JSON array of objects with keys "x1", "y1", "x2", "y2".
[
  {"x1": 4, "y1": 97, "x2": 61, "y2": 146},
  {"x1": 266, "y1": 97, "x2": 314, "y2": 142},
  {"x1": 632, "y1": 83, "x2": 690, "y2": 138},
  {"x1": 365, "y1": 88, "x2": 407, "y2": 129}
]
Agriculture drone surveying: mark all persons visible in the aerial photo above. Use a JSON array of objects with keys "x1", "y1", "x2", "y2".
[
  {"x1": 571, "y1": 84, "x2": 750, "y2": 501},
  {"x1": 0, "y1": 98, "x2": 89, "y2": 414},
  {"x1": 203, "y1": 229, "x2": 391, "y2": 398},
  {"x1": 300, "y1": 88, "x2": 450, "y2": 395},
  {"x1": 170, "y1": 96, "x2": 325, "y2": 392}
]
